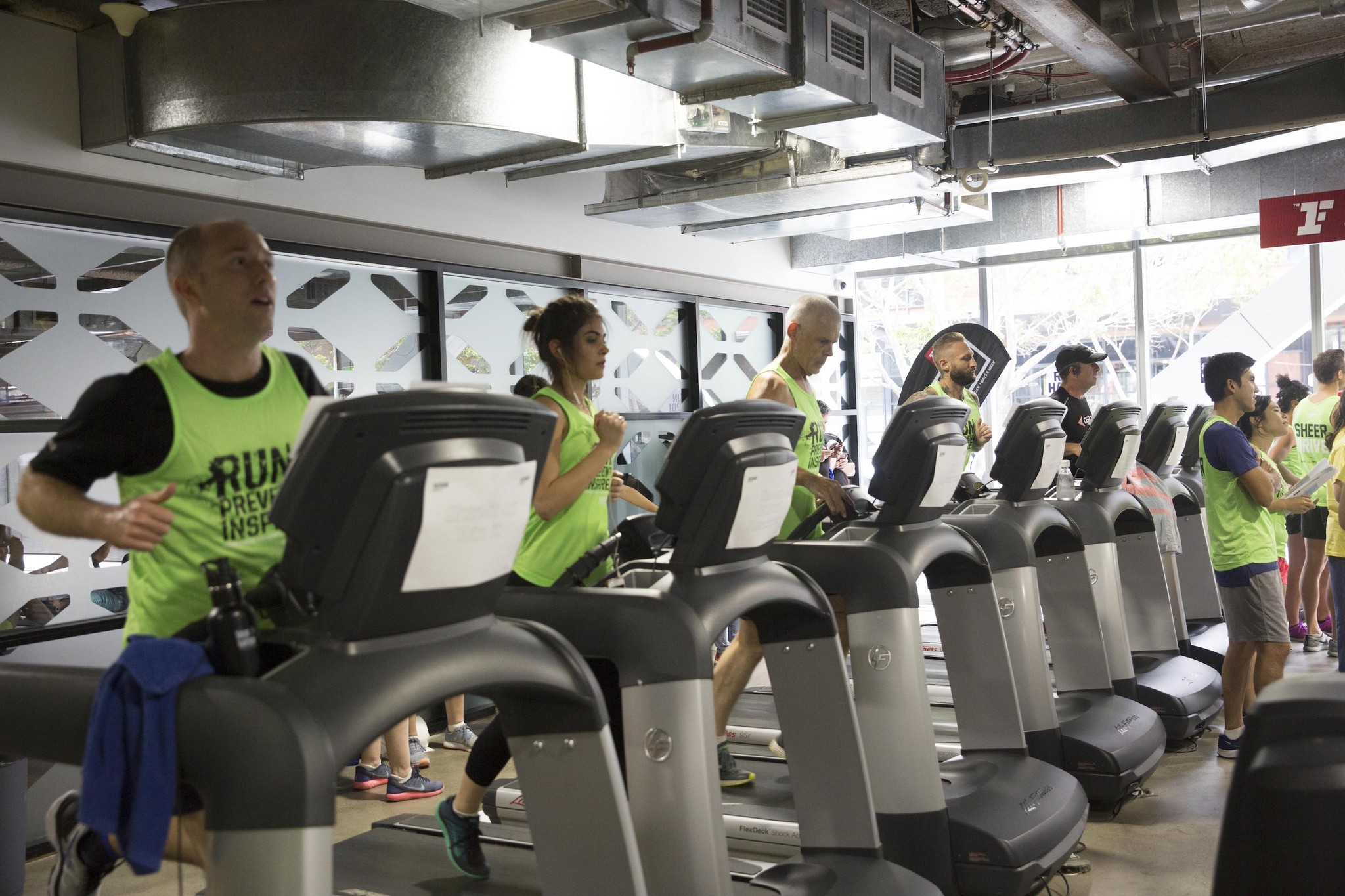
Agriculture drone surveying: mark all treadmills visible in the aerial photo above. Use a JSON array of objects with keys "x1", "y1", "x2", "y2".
[{"x1": 1, "y1": 388, "x2": 1251, "y2": 896}]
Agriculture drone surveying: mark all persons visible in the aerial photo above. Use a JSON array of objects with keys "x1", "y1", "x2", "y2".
[
  {"x1": 713, "y1": 295, "x2": 854, "y2": 785},
  {"x1": 1268, "y1": 371, "x2": 1333, "y2": 640},
  {"x1": 1324, "y1": 389, "x2": 1345, "y2": 672},
  {"x1": 1291, "y1": 349, "x2": 1345, "y2": 657},
  {"x1": 1338, "y1": 484, "x2": 1345, "y2": 531},
  {"x1": 1046, "y1": 345, "x2": 1107, "y2": 482},
  {"x1": 16, "y1": 223, "x2": 331, "y2": 896},
  {"x1": 817, "y1": 400, "x2": 856, "y2": 498},
  {"x1": 1200, "y1": 352, "x2": 1289, "y2": 758},
  {"x1": 439, "y1": 296, "x2": 626, "y2": 884},
  {"x1": 1238, "y1": 396, "x2": 1318, "y2": 719},
  {"x1": 900, "y1": 333, "x2": 993, "y2": 472},
  {"x1": 353, "y1": 376, "x2": 739, "y2": 800}
]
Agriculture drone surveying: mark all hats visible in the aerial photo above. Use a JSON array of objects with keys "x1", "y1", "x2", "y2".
[{"x1": 1055, "y1": 345, "x2": 1108, "y2": 372}]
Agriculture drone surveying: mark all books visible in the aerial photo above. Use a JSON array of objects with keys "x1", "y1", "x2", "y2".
[{"x1": 1281, "y1": 457, "x2": 1337, "y2": 517}]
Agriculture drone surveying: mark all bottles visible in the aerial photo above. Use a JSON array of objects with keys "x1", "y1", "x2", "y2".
[
  {"x1": 202, "y1": 557, "x2": 263, "y2": 678},
  {"x1": 1056, "y1": 460, "x2": 1075, "y2": 498}
]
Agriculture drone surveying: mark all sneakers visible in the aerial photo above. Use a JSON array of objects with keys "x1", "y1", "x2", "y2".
[
  {"x1": 1317, "y1": 616, "x2": 1333, "y2": 636},
  {"x1": 443, "y1": 724, "x2": 479, "y2": 752},
  {"x1": 718, "y1": 740, "x2": 756, "y2": 787},
  {"x1": 1303, "y1": 632, "x2": 1332, "y2": 652},
  {"x1": 435, "y1": 794, "x2": 490, "y2": 879},
  {"x1": 1242, "y1": 711, "x2": 1248, "y2": 723},
  {"x1": 1217, "y1": 733, "x2": 1243, "y2": 758},
  {"x1": 1327, "y1": 639, "x2": 1338, "y2": 657},
  {"x1": 385, "y1": 766, "x2": 444, "y2": 802},
  {"x1": 768, "y1": 733, "x2": 787, "y2": 760},
  {"x1": 44, "y1": 790, "x2": 126, "y2": 895},
  {"x1": 1289, "y1": 619, "x2": 1308, "y2": 642},
  {"x1": 354, "y1": 763, "x2": 392, "y2": 790},
  {"x1": 408, "y1": 734, "x2": 430, "y2": 767}
]
